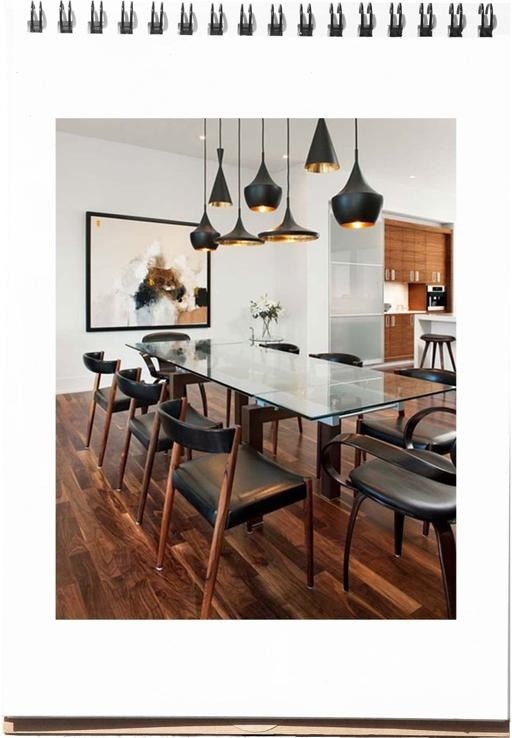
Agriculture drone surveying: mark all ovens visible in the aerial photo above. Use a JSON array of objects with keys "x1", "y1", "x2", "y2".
[{"x1": 427, "y1": 285, "x2": 446, "y2": 311}]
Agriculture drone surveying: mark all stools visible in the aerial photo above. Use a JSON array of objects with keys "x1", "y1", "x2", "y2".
[{"x1": 418, "y1": 333, "x2": 455, "y2": 372}]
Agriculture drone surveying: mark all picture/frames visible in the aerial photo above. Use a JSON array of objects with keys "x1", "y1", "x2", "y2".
[{"x1": 85, "y1": 210, "x2": 212, "y2": 331}]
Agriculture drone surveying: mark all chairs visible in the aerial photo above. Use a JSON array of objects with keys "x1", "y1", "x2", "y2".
[
  {"x1": 270, "y1": 352, "x2": 367, "y2": 469},
  {"x1": 82, "y1": 350, "x2": 161, "y2": 466},
  {"x1": 158, "y1": 396, "x2": 316, "y2": 619},
  {"x1": 320, "y1": 405, "x2": 456, "y2": 618},
  {"x1": 225, "y1": 343, "x2": 302, "y2": 448},
  {"x1": 136, "y1": 332, "x2": 211, "y2": 419},
  {"x1": 113, "y1": 364, "x2": 226, "y2": 525},
  {"x1": 354, "y1": 367, "x2": 455, "y2": 479}
]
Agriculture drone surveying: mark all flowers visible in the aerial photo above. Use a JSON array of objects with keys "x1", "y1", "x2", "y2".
[{"x1": 248, "y1": 293, "x2": 284, "y2": 338}]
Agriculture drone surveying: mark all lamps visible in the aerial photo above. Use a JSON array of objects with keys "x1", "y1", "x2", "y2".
[{"x1": 189, "y1": 120, "x2": 384, "y2": 253}]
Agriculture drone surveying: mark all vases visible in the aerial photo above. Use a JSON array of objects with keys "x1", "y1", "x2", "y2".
[{"x1": 262, "y1": 319, "x2": 272, "y2": 339}]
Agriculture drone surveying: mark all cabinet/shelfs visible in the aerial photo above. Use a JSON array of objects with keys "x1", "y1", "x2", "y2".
[
  {"x1": 385, "y1": 219, "x2": 450, "y2": 283},
  {"x1": 384, "y1": 311, "x2": 427, "y2": 362}
]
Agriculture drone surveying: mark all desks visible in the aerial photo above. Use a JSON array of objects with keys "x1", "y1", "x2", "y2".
[
  {"x1": 249, "y1": 336, "x2": 284, "y2": 348},
  {"x1": 126, "y1": 341, "x2": 455, "y2": 535},
  {"x1": 411, "y1": 313, "x2": 455, "y2": 371}
]
